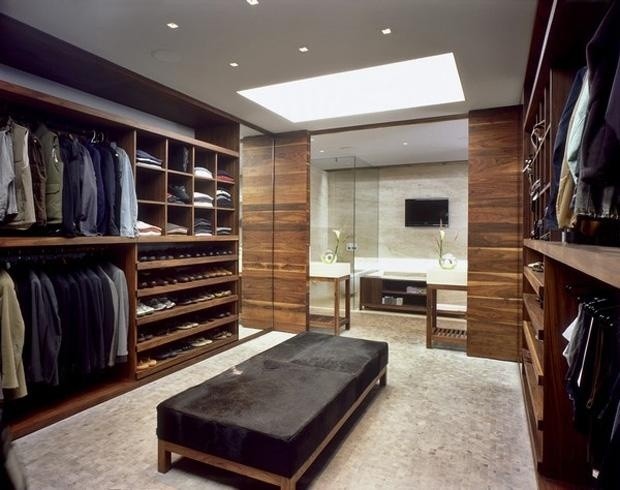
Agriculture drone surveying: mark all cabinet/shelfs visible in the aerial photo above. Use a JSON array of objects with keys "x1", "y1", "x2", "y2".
[
  {"x1": 2, "y1": 85, "x2": 137, "y2": 239},
  {"x1": 522, "y1": 2, "x2": 541, "y2": 488},
  {"x1": 2, "y1": 11, "x2": 238, "y2": 153},
  {"x1": 1, "y1": 239, "x2": 137, "y2": 444},
  {"x1": 136, "y1": 239, "x2": 239, "y2": 385},
  {"x1": 542, "y1": 2, "x2": 620, "y2": 490},
  {"x1": 137, "y1": 125, "x2": 240, "y2": 239},
  {"x1": 359, "y1": 272, "x2": 426, "y2": 314}
]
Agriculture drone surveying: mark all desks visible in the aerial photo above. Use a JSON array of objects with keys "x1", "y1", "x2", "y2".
[
  {"x1": 425, "y1": 284, "x2": 467, "y2": 351},
  {"x1": 309, "y1": 275, "x2": 350, "y2": 336}
]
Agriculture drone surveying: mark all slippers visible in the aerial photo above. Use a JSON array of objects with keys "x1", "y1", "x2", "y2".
[{"x1": 137, "y1": 312, "x2": 232, "y2": 370}]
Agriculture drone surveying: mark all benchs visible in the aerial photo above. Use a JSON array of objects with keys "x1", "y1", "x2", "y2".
[{"x1": 156, "y1": 330, "x2": 388, "y2": 489}]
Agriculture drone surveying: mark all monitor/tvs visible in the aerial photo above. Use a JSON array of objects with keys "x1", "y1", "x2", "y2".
[{"x1": 405, "y1": 198, "x2": 448, "y2": 226}]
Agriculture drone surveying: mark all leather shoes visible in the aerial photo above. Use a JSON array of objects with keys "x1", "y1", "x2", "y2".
[
  {"x1": 139, "y1": 246, "x2": 232, "y2": 261},
  {"x1": 138, "y1": 266, "x2": 233, "y2": 288}
]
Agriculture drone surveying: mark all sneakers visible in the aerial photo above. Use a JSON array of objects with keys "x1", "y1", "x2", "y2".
[{"x1": 137, "y1": 286, "x2": 232, "y2": 317}]
